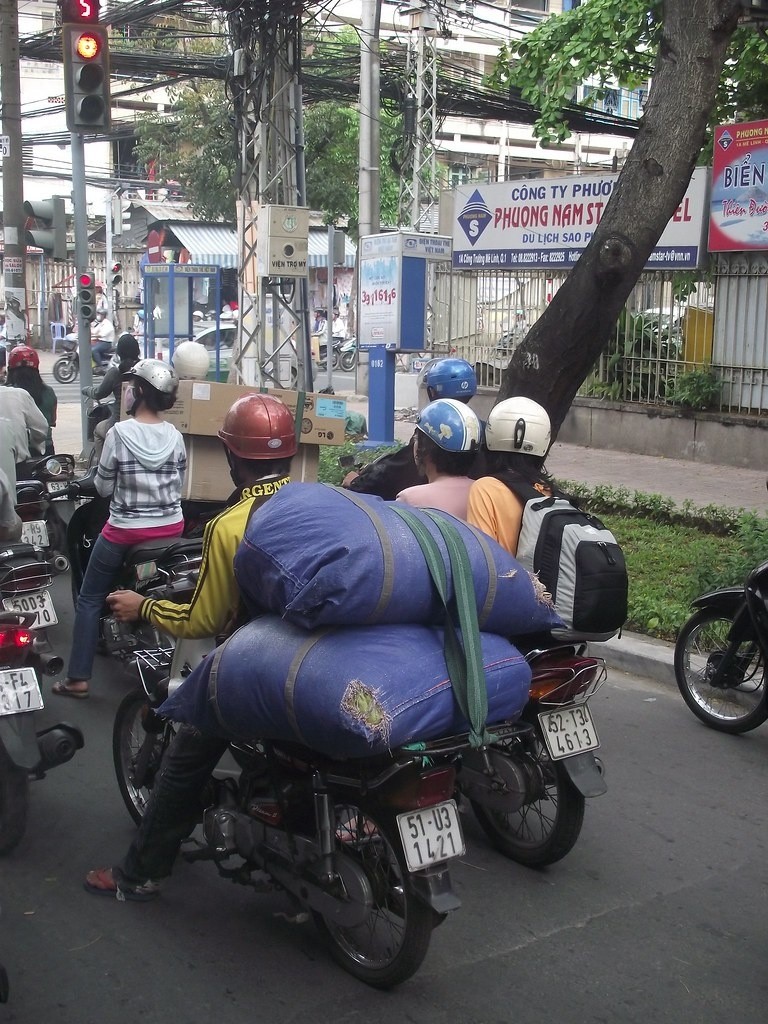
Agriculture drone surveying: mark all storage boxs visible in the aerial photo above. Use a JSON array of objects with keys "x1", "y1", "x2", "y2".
[
  {"x1": 180, "y1": 434, "x2": 319, "y2": 506},
  {"x1": 119, "y1": 379, "x2": 349, "y2": 449}
]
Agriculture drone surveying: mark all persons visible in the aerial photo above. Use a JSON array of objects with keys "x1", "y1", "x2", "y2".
[
  {"x1": 95, "y1": 281, "x2": 108, "y2": 310},
  {"x1": 82, "y1": 393, "x2": 297, "y2": 900},
  {"x1": 465, "y1": 395, "x2": 553, "y2": 557},
  {"x1": 53, "y1": 359, "x2": 187, "y2": 699},
  {"x1": 81, "y1": 335, "x2": 140, "y2": 466},
  {"x1": 313, "y1": 308, "x2": 345, "y2": 354},
  {"x1": 512, "y1": 310, "x2": 526, "y2": 335},
  {"x1": 171, "y1": 342, "x2": 210, "y2": 380},
  {"x1": 0, "y1": 315, "x2": 58, "y2": 545},
  {"x1": 130, "y1": 310, "x2": 149, "y2": 343},
  {"x1": 192, "y1": 299, "x2": 237, "y2": 321},
  {"x1": 397, "y1": 398, "x2": 480, "y2": 525},
  {"x1": 92, "y1": 309, "x2": 115, "y2": 373},
  {"x1": 343, "y1": 359, "x2": 476, "y2": 499}
]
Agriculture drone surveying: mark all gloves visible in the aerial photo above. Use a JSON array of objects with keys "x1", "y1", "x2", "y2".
[{"x1": 82, "y1": 386, "x2": 89, "y2": 395}]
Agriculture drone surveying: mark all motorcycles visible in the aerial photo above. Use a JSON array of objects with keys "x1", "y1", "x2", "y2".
[
  {"x1": 52, "y1": 336, "x2": 115, "y2": 384},
  {"x1": 336, "y1": 453, "x2": 617, "y2": 868},
  {"x1": 0, "y1": 393, "x2": 227, "y2": 861},
  {"x1": 97, "y1": 552, "x2": 537, "y2": 993}
]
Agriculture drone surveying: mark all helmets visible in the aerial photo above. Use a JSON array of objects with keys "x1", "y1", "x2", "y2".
[
  {"x1": 313, "y1": 307, "x2": 323, "y2": 312},
  {"x1": 333, "y1": 309, "x2": 339, "y2": 315},
  {"x1": 9, "y1": 346, "x2": 39, "y2": 370},
  {"x1": 485, "y1": 396, "x2": 551, "y2": 457},
  {"x1": 205, "y1": 310, "x2": 216, "y2": 316},
  {"x1": 193, "y1": 311, "x2": 203, "y2": 318},
  {"x1": 426, "y1": 359, "x2": 477, "y2": 402},
  {"x1": 137, "y1": 310, "x2": 145, "y2": 319},
  {"x1": 121, "y1": 358, "x2": 179, "y2": 392},
  {"x1": 218, "y1": 393, "x2": 297, "y2": 459},
  {"x1": 95, "y1": 281, "x2": 102, "y2": 288},
  {"x1": 415, "y1": 396, "x2": 482, "y2": 453},
  {"x1": 172, "y1": 340, "x2": 209, "y2": 379},
  {"x1": 97, "y1": 308, "x2": 108, "y2": 315}
]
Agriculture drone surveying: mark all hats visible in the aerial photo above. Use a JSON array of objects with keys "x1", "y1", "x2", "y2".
[{"x1": 117, "y1": 334, "x2": 140, "y2": 359}]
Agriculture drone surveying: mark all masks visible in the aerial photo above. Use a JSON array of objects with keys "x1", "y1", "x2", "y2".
[
  {"x1": 125, "y1": 386, "x2": 136, "y2": 411},
  {"x1": 207, "y1": 317, "x2": 212, "y2": 321},
  {"x1": 97, "y1": 316, "x2": 102, "y2": 321}
]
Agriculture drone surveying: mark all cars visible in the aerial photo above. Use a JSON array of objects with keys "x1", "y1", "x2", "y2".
[
  {"x1": 640, "y1": 306, "x2": 686, "y2": 348},
  {"x1": 105, "y1": 320, "x2": 319, "y2": 389}
]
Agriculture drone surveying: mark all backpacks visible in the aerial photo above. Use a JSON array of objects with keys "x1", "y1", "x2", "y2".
[{"x1": 495, "y1": 472, "x2": 628, "y2": 642}]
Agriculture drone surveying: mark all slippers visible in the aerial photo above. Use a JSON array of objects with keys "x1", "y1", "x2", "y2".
[
  {"x1": 334, "y1": 825, "x2": 379, "y2": 843},
  {"x1": 52, "y1": 680, "x2": 89, "y2": 699},
  {"x1": 84, "y1": 866, "x2": 160, "y2": 901}
]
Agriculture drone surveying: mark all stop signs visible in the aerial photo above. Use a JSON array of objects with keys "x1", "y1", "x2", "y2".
[{"x1": 146, "y1": 229, "x2": 162, "y2": 263}]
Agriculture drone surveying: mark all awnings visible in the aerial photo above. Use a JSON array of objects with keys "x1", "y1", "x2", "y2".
[{"x1": 169, "y1": 224, "x2": 357, "y2": 269}]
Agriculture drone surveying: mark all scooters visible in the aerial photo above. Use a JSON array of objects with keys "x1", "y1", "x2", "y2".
[
  {"x1": 675, "y1": 553, "x2": 768, "y2": 735},
  {"x1": 311, "y1": 334, "x2": 358, "y2": 374}
]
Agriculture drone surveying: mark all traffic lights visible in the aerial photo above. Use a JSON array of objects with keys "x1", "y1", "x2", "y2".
[
  {"x1": 78, "y1": 271, "x2": 97, "y2": 323},
  {"x1": 58, "y1": 0, "x2": 104, "y2": 24},
  {"x1": 106, "y1": 260, "x2": 123, "y2": 288},
  {"x1": 24, "y1": 197, "x2": 66, "y2": 260},
  {"x1": 114, "y1": 199, "x2": 132, "y2": 236},
  {"x1": 61, "y1": 20, "x2": 116, "y2": 136}
]
